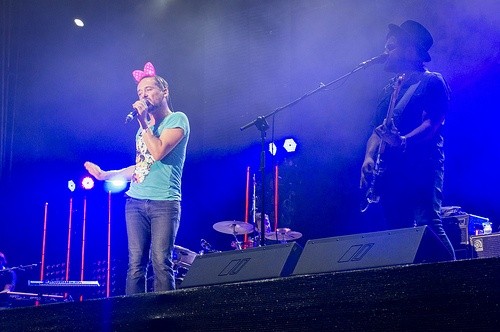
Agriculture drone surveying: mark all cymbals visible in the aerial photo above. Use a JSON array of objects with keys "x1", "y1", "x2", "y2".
[
  {"x1": 212, "y1": 218, "x2": 255, "y2": 237},
  {"x1": 263, "y1": 229, "x2": 303, "y2": 242}
]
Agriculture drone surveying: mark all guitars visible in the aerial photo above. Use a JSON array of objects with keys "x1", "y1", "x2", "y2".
[{"x1": 353, "y1": 69, "x2": 407, "y2": 214}]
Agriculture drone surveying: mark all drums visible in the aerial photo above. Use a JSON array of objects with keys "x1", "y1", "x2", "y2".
[{"x1": 170, "y1": 242, "x2": 199, "y2": 266}]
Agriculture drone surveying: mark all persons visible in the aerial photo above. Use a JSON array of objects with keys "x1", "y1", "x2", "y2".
[
  {"x1": 0, "y1": 252, "x2": 16, "y2": 308},
  {"x1": 83, "y1": 61, "x2": 190, "y2": 296},
  {"x1": 359, "y1": 20, "x2": 455, "y2": 264}
]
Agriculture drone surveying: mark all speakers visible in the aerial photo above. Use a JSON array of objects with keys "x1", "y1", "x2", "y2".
[
  {"x1": 292, "y1": 225, "x2": 456, "y2": 275},
  {"x1": 179, "y1": 242, "x2": 304, "y2": 289}
]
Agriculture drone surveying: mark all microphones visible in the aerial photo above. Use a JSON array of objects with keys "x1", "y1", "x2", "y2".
[
  {"x1": 125, "y1": 99, "x2": 148, "y2": 122},
  {"x1": 359, "y1": 54, "x2": 387, "y2": 67}
]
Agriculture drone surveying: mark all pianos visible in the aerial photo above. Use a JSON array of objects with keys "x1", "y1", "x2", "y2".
[{"x1": 27, "y1": 279, "x2": 101, "y2": 291}]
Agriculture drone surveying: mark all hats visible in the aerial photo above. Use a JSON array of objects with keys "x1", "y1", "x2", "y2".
[{"x1": 389, "y1": 19, "x2": 433, "y2": 62}]
